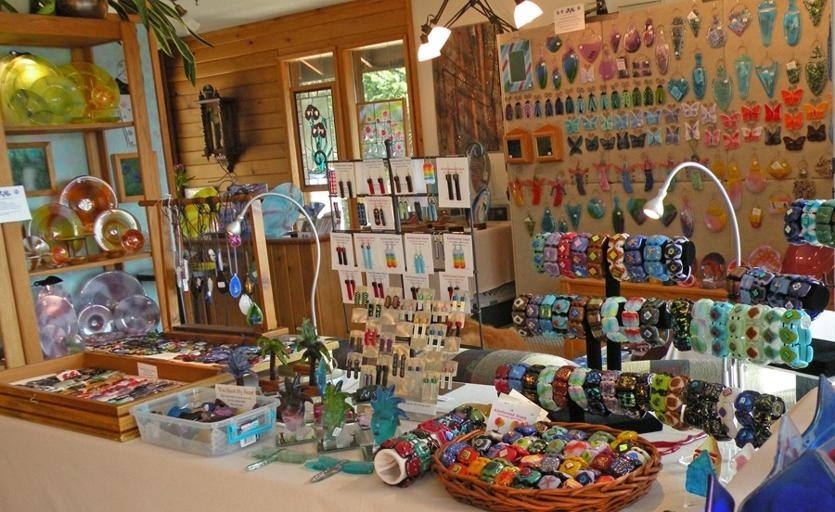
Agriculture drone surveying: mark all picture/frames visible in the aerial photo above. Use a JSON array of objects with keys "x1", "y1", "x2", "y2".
[
  {"x1": 6, "y1": 140, "x2": 58, "y2": 197},
  {"x1": 112, "y1": 150, "x2": 145, "y2": 204}
]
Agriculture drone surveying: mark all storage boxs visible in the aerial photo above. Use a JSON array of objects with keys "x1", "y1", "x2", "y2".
[{"x1": 131, "y1": 384, "x2": 280, "y2": 457}]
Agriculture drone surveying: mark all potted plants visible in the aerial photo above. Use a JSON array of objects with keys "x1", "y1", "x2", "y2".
[
  {"x1": 54, "y1": 0, "x2": 214, "y2": 86},
  {"x1": 295, "y1": 322, "x2": 332, "y2": 396},
  {"x1": 277, "y1": 377, "x2": 310, "y2": 430},
  {"x1": 254, "y1": 335, "x2": 291, "y2": 395}
]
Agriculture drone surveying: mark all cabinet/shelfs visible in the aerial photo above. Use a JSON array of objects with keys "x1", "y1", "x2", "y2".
[{"x1": 0, "y1": 0, "x2": 173, "y2": 383}]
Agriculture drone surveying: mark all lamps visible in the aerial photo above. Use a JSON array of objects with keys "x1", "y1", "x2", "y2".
[
  {"x1": 411, "y1": 0, "x2": 543, "y2": 68},
  {"x1": 643, "y1": 160, "x2": 742, "y2": 267},
  {"x1": 224, "y1": 191, "x2": 326, "y2": 342}
]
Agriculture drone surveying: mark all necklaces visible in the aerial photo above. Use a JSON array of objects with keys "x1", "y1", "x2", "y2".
[{"x1": 163, "y1": 192, "x2": 263, "y2": 325}]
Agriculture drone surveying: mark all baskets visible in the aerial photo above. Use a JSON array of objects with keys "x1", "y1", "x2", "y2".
[{"x1": 432, "y1": 421, "x2": 664, "y2": 511}]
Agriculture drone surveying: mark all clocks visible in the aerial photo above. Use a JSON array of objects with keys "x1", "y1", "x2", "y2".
[{"x1": 193, "y1": 84, "x2": 246, "y2": 173}]
